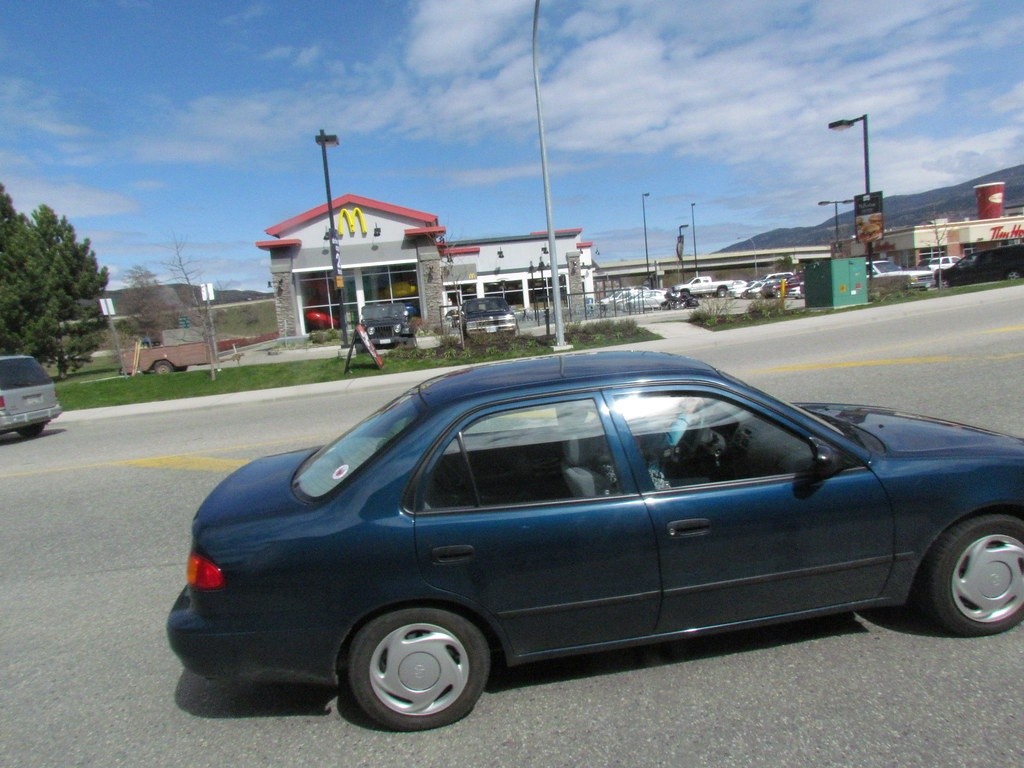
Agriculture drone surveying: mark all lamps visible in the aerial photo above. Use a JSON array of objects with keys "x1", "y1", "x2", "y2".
[
  {"x1": 497, "y1": 246, "x2": 504, "y2": 258},
  {"x1": 542, "y1": 243, "x2": 549, "y2": 253},
  {"x1": 267, "y1": 279, "x2": 283, "y2": 288},
  {"x1": 593, "y1": 244, "x2": 599, "y2": 254},
  {"x1": 579, "y1": 248, "x2": 583, "y2": 254},
  {"x1": 324, "y1": 226, "x2": 330, "y2": 239},
  {"x1": 374, "y1": 222, "x2": 381, "y2": 236}
]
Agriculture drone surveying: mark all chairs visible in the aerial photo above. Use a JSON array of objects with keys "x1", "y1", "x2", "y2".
[{"x1": 561, "y1": 432, "x2": 612, "y2": 496}]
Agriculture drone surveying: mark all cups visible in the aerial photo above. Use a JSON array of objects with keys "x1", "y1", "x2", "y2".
[{"x1": 973, "y1": 182, "x2": 1005, "y2": 218}]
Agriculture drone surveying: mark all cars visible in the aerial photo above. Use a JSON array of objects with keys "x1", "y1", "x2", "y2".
[
  {"x1": 598, "y1": 254, "x2": 963, "y2": 310},
  {"x1": 941, "y1": 240, "x2": 1024, "y2": 287},
  {"x1": 0, "y1": 355, "x2": 63, "y2": 438},
  {"x1": 458, "y1": 295, "x2": 520, "y2": 341},
  {"x1": 352, "y1": 301, "x2": 419, "y2": 356},
  {"x1": 163, "y1": 348, "x2": 1023, "y2": 735}
]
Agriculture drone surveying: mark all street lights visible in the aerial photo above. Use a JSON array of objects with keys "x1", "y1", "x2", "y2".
[
  {"x1": 817, "y1": 199, "x2": 855, "y2": 256},
  {"x1": 314, "y1": 129, "x2": 352, "y2": 351},
  {"x1": 738, "y1": 236, "x2": 758, "y2": 279},
  {"x1": 691, "y1": 202, "x2": 699, "y2": 278},
  {"x1": 641, "y1": 191, "x2": 652, "y2": 291},
  {"x1": 828, "y1": 113, "x2": 873, "y2": 300}
]
